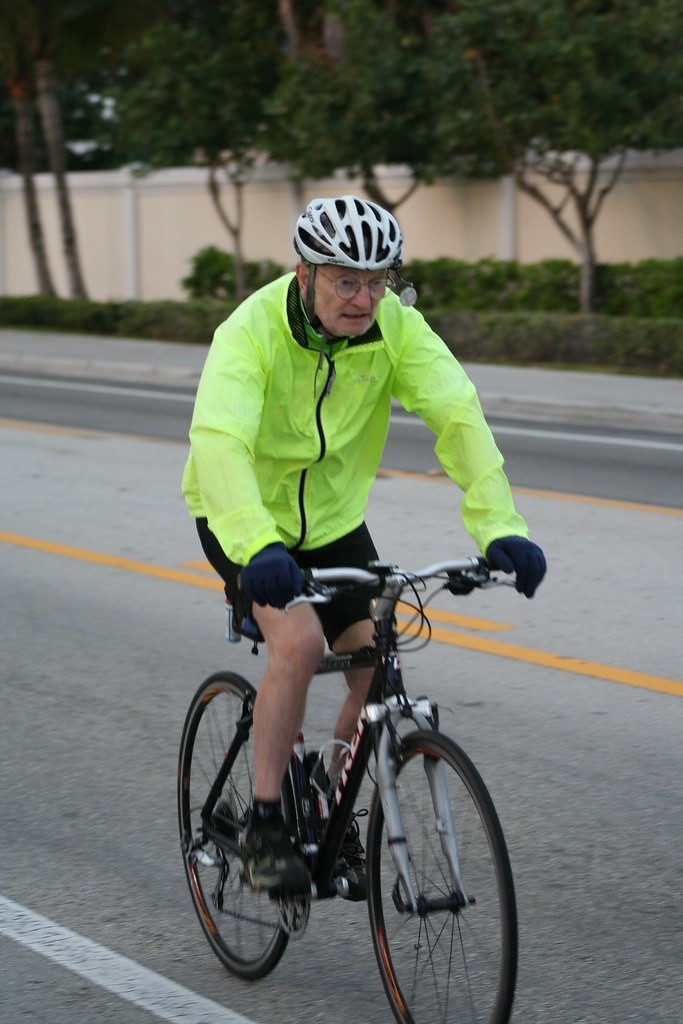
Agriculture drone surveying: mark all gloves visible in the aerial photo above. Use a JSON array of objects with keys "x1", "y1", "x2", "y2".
[
  {"x1": 240, "y1": 542, "x2": 304, "y2": 610},
  {"x1": 485, "y1": 535, "x2": 547, "y2": 599}
]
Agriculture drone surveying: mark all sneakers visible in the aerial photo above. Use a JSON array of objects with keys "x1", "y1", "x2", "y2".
[
  {"x1": 303, "y1": 751, "x2": 368, "y2": 901},
  {"x1": 240, "y1": 823, "x2": 310, "y2": 892}
]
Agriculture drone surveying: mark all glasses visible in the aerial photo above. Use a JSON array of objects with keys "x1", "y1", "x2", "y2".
[{"x1": 316, "y1": 269, "x2": 396, "y2": 300}]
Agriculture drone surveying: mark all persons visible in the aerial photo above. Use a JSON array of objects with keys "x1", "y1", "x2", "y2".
[{"x1": 180, "y1": 195, "x2": 547, "y2": 902}]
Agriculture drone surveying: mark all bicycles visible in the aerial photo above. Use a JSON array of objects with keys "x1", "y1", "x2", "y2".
[{"x1": 174, "y1": 551, "x2": 521, "y2": 1024}]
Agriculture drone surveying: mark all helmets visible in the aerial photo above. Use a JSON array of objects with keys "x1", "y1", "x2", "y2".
[{"x1": 293, "y1": 194, "x2": 405, "y2": 270}]
